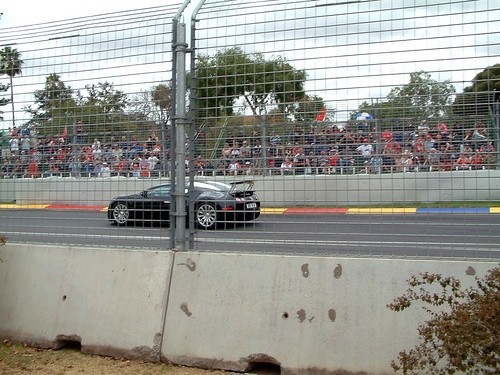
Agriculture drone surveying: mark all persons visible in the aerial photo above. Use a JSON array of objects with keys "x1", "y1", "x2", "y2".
[{"x1": 0, "y1": 119, "x2": 497, "y2": 177}]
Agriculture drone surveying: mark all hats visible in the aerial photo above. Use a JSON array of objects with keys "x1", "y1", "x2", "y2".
[{"x1": 363, "y1": 139, "x2": 368, "y2": 141}]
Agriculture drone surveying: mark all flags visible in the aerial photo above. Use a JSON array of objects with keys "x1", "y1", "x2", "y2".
[
  {"x1": 316, "y1": 106, "x2": 327, "y2": 121},
  {"x1": 62, "y1": 126, "x2": 67, "y2": 139}
]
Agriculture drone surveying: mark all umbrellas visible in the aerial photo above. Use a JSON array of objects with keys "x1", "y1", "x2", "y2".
[{"x1": 353, "y1": 112, "x2": 373, "y2": 120}]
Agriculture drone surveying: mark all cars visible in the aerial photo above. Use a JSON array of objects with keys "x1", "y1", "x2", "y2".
[{"x1": 108, "y1": 178, "x2": 261, "y2": 230}]
